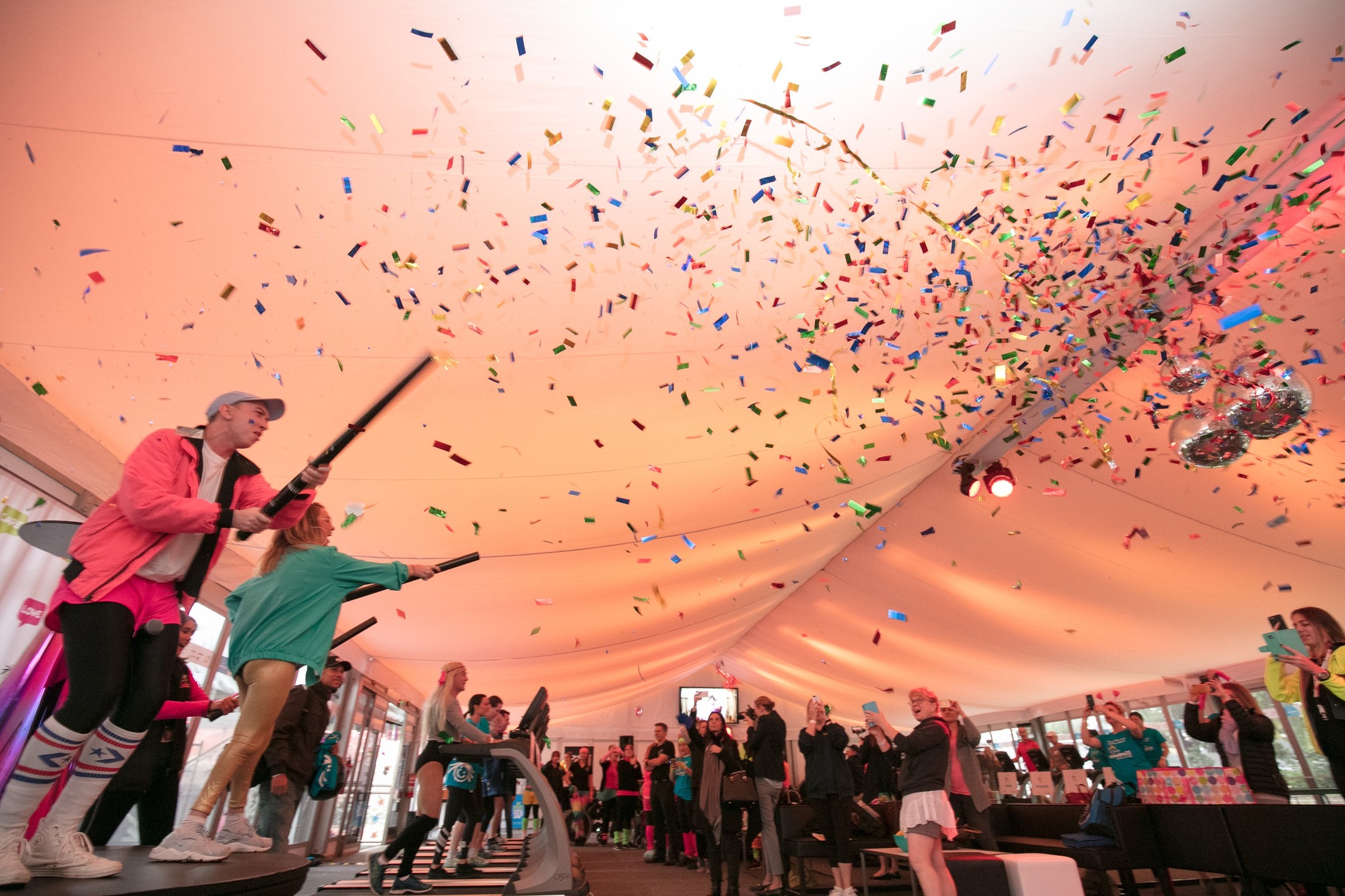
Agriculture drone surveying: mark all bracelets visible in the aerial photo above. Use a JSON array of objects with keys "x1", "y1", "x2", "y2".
[
  {"x1": 683, "y1": 766, "x2": 687, "y2": 771},
  {"x1": 809, "y1": 720, "x2": 816, "y2": 724},
  {"x1": 646, "y1": 759, "x2": 650, "y2": 765},
  {"x1": 1082, "y1": 720, "x2": 1087, "y2": 723},
  {"x1": 825, "y1": 719, "x2": 831, "y2": 723},
  {"x1": 411, "y1": 565, "x2": 415, "y2": 576},
  {"x1": 490, "y1": 737, "x2": 494, "y2": 743},
  {"x1": 877, "y1": 738, "x2": 886, "y2": 746}
]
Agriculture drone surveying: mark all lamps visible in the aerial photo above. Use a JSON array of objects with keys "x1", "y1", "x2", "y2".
[
  {"x1": 954, "y1": 462, "x2": 982, "y2": 499},
  {"x1": 983, "y1": 459, "x2": 1016, "y2": 498},
  {"x1": 1154, "y1": 312, "x2": 1314, "y2": 471}
]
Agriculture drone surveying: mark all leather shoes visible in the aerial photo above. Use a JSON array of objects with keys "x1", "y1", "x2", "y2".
[
  {"x1": 890, "y1": 871, "x2": 900, "y2": 878},
  {"x1": 871, "y1": 873, "x2": 891, "y2": 879}
]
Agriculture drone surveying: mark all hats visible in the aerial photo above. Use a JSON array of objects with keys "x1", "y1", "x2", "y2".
[
  {"x1": 325, "y1": 656, "x2": 352, "y2": 671},
  {"x1": 1044, "y1": 732, "x2": 1056, "y2": 736},
  {"x1": 207, "y1": 392, "x2": 285, "y2": 421}
]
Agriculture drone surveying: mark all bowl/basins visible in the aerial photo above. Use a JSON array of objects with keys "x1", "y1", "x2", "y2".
[{"x1": 893, "y1": 834, "x2": 908, "y2": 853}]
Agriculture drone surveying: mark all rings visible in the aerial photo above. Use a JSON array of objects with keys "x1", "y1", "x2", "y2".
[
  {"x1": 224, "y1": 706, "x2": 228, "y2": 708},
  {"x1": 424, "y1": 578, "x2": 428, "y2": 580},
  {"x1": 434, "y1": 566, "x2": 436, "y2": 571}
]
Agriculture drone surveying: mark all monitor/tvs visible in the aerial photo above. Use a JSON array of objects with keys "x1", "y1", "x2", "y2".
[
  {"x1": 1059, "y1": 748, "x2": 1084, "y2": 768},
  {"x1": 1027, "y1": 748, "x2": 1049, "y2": 771},
  {"x1": 995, "y1": 751, "x2": 1016, "y2": 771},
  {"x1": 518, "y1": 686, "x2": 550, "y2": 753},
  {"x1": 679, "y1": 687, "x2": 739, "y2": 725}
]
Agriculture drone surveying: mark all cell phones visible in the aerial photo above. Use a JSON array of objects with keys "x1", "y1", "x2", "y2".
[
  {"x1": 1086, "y1": 694, "x2": 1095, "y2": 710},
  {"x1": 670, "y1": 758, "x2": 677, "y2": 768},
  {"x1": 1269, "y1": 615, "x2": 1287, "y2": 631},
  {"x1": 1193, "y1": 684, "x2": 1212, "y2": 695},
  {"x1": 812, "y1": 695, "x2": 820, "y2": 703},
  {"x1": 862, "y1": 701, "x2": 880, "y2": 727}
]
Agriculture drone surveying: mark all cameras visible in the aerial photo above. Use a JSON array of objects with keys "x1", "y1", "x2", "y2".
[{"x1": 738, "y1": 705, "x2": 757, "y2": 720}]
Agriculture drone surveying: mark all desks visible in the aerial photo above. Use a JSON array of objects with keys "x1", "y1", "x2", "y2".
[{"x1": 856, "y1": 846, "x2": 1014, "y2": 896}]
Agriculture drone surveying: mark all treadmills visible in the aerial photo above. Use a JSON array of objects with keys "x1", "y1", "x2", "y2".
[{"x1": 310, "y1": 685, "x2": 595, "y2": 896}]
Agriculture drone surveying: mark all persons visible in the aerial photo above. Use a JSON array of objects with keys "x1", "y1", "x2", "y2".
[
  {"x1": 844, "y1": 734, "x2": 901, "y2": 880},
  {"x1": 366, "y1": 662, "x2": 502, "y2": 895},
  {"x1": 252, "y1": 655, "x2": 352, "y2": 853},
  {"x1": 798, "y1": 698, "x2": 857, "y2": 896},
  {"x1": 864, "y1": 687, "x2": 959, "y2": 896},
  {"x1": 78, "y1": 615, "x2": 240, "y2": 846},
  {"x1": 0, "y1": 391, "x2": 332, "y2": 885},
  {"x1": 521, "y1": 744, "x2": 644, "y2": 851},
  {"x1": 940, "y1": 699, "x2": 999, "y2": 852},
  {"x1": 644, "y1": 717, "x2": 711, "y2": 874},
  {"x1": 1081, "y1": 702, "x2": 1169, "y2": 804},
  {"x1": 148, "y1": 503, "x2": 440, "y2": 863},
  {"x1": 1011, "y1": 727, "x2": 1071, "y2": 771},
  {"x1": 1263, "y1": 606, "x2": 1345, "y2": 800},
  {"x1": 428, "y1": 694, "x2": 517, "y2": 879},
  {"x1": 1184, "y1": 669, "x2": 1290, "y2": 799},
  {"x1": 686, "y1": 694, "x2": 791, "y2": 896}
]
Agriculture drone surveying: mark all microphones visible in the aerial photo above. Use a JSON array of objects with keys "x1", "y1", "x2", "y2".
[{"x1": 130, "y1": 619, "x2": 163, "y2": 640}]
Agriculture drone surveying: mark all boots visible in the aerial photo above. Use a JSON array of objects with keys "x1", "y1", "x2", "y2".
[
  {"x1": 708, "y1": 884, "x2": 720, "y2": 896},
  {"x1": 726, "y1": 882, "x2": 739, "y2": 896}
]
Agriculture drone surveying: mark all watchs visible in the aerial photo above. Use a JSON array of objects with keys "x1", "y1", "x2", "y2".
[{"x1": 1318, "y1": 669, "x2": 1331, "y2": 681}]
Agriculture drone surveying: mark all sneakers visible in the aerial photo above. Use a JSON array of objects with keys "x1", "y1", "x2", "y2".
[
  {"x1": 149, "y1": 825, "x2": 232, "y2": 862},
  {"x1": 0, "y1": 828, "x2": 33, "y2": 884},
  {"x1": 389, "y1": 875, "x2": 433, "y2": 894},
  {"x1": 365, "y1": 851, "x2": 389, "y2": 895},
  {"x1": 745, "y1": 861, "x2": 761, "y2": 870},
  {"x1": 828, "y1": 885, "x2": 859, "y2": 896},
  {"x1": 23, "y1": 818, "x2": 123, "y2": 878},
  {"x1": 466, "y1": 834, "x2": 507, "y2": 866},
  {"x1": 216, "y1": 819, "x2": 273, "y2": 852}
]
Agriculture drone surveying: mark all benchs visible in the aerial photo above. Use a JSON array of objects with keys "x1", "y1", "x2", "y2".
[{"x1": 774, "y1": 799, "x2": 1175, "y2": 896}]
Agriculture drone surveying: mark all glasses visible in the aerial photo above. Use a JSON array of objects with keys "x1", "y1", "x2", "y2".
[{"x1": 908, "y1": 698, "x2": 931, "y2": 705}]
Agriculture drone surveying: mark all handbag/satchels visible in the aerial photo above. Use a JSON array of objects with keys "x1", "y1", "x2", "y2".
[{"x1": 721, "y1": 761, "x2": 758, "y2": 808}]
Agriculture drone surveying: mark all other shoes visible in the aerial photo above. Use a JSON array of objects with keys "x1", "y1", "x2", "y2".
[
  {"x1": 758, "y1": 887, "x2": 783, "y2": 896},
  {"x1": 751, "y1": 884, "x2": 772, "y2": 890},
  {"x1": 456, "y1": 863, "x2": 482, "y2": 877},
  {"x1": 427, "y1": 869, "x2": 455, "y2": 879},
  {"x1": 444, "y1": 857, "x2": 460, "y2": 867},
  {"x1": 644, "y1": 849, "x2": 710, "y2": 874},
  {"x1": 598, "y1": 839, "x2": 638, "y2": 851}
]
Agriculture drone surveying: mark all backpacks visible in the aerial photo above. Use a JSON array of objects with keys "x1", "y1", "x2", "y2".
[{"x1": 1077, "y1": 780, "x2": 1138, "y2": 839}]
